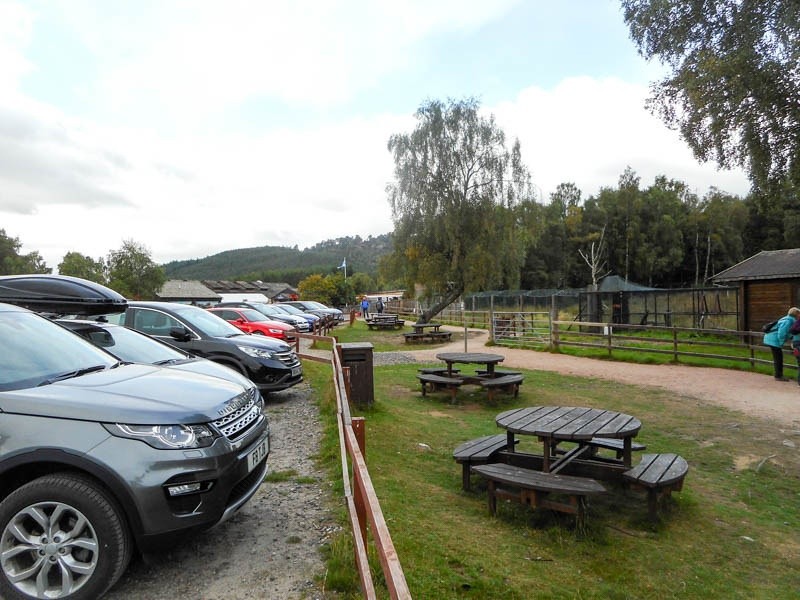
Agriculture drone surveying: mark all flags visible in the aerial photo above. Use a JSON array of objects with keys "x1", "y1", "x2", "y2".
[{"x1": 337, "y1": 260, "x2": 345, "y2": 269}]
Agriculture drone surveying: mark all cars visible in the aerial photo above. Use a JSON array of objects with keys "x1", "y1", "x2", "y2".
[
  {"x1": 0, "y1": 275, "x2": 266, "y2": 411},
  {"x1": 62, "y1": 300, "x2": 304, "y2": 395},
  {"x1": 205, "y1": 301, "x2": 345, "y2": 343}
]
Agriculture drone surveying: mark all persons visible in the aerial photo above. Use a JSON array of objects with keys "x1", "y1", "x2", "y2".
[
  {"x1": 791, "y1": 320, "x2": 800, "y2": 388},
  {"x1": 765, "y1": 307, "x2": 800, "y2": 382},
  {"x1": 376, "y1": 297, "x2": 385, "y2": 314},
  {"x1": 361, "y1": 297, "x2": 370, "y2": 319}
]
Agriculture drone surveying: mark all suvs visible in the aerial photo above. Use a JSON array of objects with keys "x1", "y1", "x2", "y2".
[{"x1": 0, "y1": 304, "x2": 274, "y2": 600}]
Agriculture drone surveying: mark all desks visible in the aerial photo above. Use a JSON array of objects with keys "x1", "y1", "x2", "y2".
[
  {"x1": 495, "y1": 406, "x2": 641, "y2": 497},
  {"x1": 412, "y1": 323, "x2": 441, "y2": 343},
  {"x1": 437, "y1": 351, "x2": 505, "y2": 404},
  {"x1": 370, "y1": 313, "x2": 398, "y2": 322}
]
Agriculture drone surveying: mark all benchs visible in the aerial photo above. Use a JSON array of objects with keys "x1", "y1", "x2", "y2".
[
  {"x1": 417, "y1": 368, "x2": 525, "y2": 404},
  {"x1": 402, "y1": 332, "x2": 452, "y2": 344},
  {"x1": 366, "y1": 318, "x2": 405, "y2": 328},
  {"x1": 453, "y1": 434, "x2": 689, "y2": 525}
]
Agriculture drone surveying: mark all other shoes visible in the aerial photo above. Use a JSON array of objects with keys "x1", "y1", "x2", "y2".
[{"x1": 775, "y1": 378, "x2": 789, "y2": 381}]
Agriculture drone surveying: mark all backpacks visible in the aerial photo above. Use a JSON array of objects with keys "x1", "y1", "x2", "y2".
[
  {"x1": 762, "y1": 319, "x2": 792, "y2": 333},
  {"x1": 377, "y1": 302, "x2": 381, "y2": 308}
]
